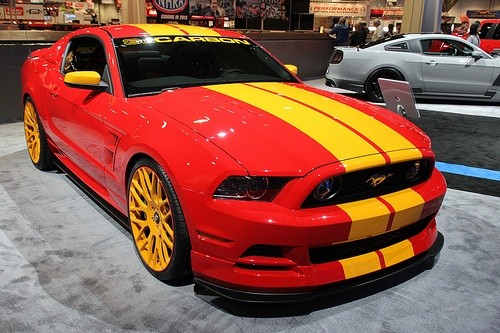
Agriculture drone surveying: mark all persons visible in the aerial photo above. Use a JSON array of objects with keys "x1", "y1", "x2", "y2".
[
  {"x1": 385, "y1": 21, "x2": 397, "y2": 41},
  {"x1": 326, "y1": 16, "x2": 350, "y2": 45},
  {"x1": 371, "y1": 17, "x2": 386, "y2": 45},
  {"x1": 465, "y1": 19, "x2": 483, "y2": 47},
  {"x1": 452, "y1": 20, "x2": 470, "y2": 37},
  {"x1": 348, "y1": 20, "x2": 371, "y2": 47}
]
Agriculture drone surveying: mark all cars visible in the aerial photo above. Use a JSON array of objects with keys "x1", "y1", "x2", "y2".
[
  {"x1": 479, "y1": 19, "x2": 500, "y2": 56},
  {"x1": 21, "y1": 23, "x2": 447, "y2": 319},
  {"x1": 324, "y1": 33, "x2": 497, "y2": 103}
]
